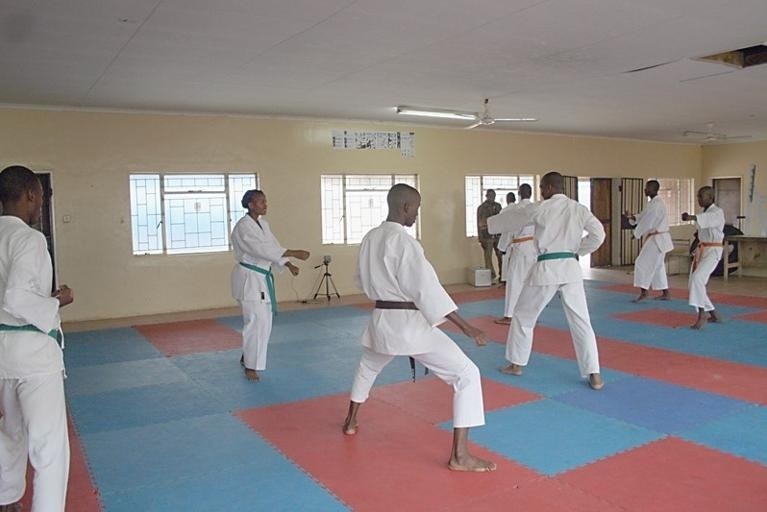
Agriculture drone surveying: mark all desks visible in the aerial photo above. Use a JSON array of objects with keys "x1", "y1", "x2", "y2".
[{"x1": 723, "y1": 234, "x2": 766, "y2": 281}]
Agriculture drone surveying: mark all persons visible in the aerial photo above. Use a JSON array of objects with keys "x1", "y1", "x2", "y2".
[
  {"x1": 0, "y1": 165, "x2": 73, "y2": 512},
  {"x1": 495, "y1": 192, "x2": 516, "y2": 287},
  {"x1": 476, "y1": 190, "x2": 503, "y2": 284},
  {"x1": 228, "y1": 188, "x2": 309, "y2": 380},
  {"x1": 625, "y1": 179, "x2": 676, "y2": 304},
  {"x1": 342, "y1": 182, "x2": 498, "y2": 474},
  {"x1": 478, "y1": 170, "x2": 608, "y2": 390},
  {"x1": 680, "y1": 185, "x2": 727, "y2": 329},
  {"x1": 495, "y1": 183, "x2": 536, "y2": 325}
]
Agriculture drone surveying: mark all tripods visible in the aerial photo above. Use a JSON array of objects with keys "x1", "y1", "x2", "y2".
[{"x1": 314, "y1": 256, "x2": 340, "y2": 301}]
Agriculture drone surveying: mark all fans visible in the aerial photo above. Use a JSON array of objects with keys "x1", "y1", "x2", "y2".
[
  {"x1": 683, "y1": 121, "x2": 753, "y2": 144},
  {"x1": 454, "y1": 98, "x2": 539, "y2": 131}
]
